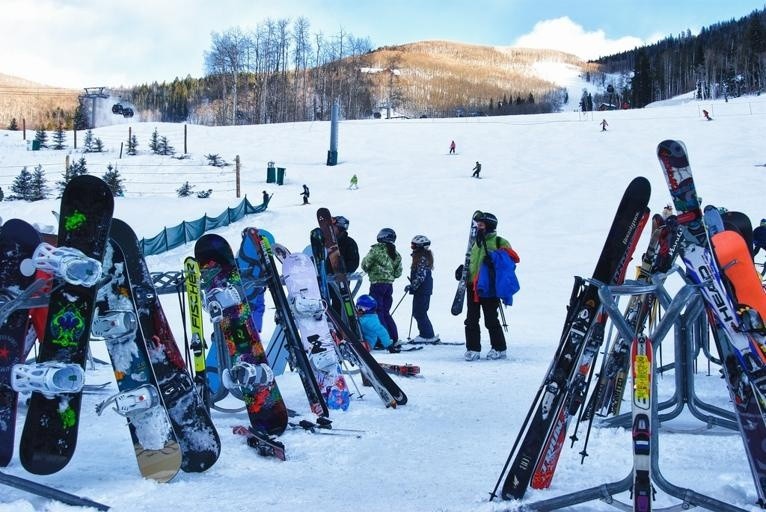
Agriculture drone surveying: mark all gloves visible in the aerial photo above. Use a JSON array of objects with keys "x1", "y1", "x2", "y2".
[
  {"x1": 404, "y1": 284, "x2": 418, "y2": 294},
  {"x1": 455, "y1": 264, "x2": 463, "y2": 280},
  {"x1": 388, "y1": 344, "x2": 401, "y2": 353}
]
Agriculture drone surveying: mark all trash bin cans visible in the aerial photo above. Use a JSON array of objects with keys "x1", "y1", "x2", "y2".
[
  {"x1": 267, "y1": 168, "x2": 275, "y2": 183},
  {"x1": 277, "y1": 168, "x2": 286, "y2": 185}
]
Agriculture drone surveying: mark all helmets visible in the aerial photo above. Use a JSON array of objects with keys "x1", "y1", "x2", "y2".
[
  {"x1": 333, "y1": 215, "x2": 350, "y2": 229},
  {"x1": 475, "y1": 212, "x2": 498, "y2": 224},
  {"x1": 411, "y1": 235, "x2": 431, "y2": 246},
  {"x1": 376, "y1": 228, "x2": 396, "y2": 244},
  {"x1": 355, "y1": 294, "x2": 378, "y2": 315}
]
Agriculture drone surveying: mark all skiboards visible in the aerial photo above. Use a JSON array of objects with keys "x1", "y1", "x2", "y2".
[
  {"x1": 396, "y1": 338, "x2": 464, "y2": 353},
  {"x1": 488, "y1": 139, "x2": 766, "y2": 512},
  {"x1": 308, "y1": 208, "x2": 421, "y2": 407},
  {"x1": 491, "y1": 139, "x2": 766, "y2": 510},
  {"x1": 233, "y1": 426, "x2": 284, "y2": 459},
  {"x1": 267, "y1": 258, "x2": 331, "y2": 418},
  {"x1": 184, "y1": 258, "x2": 211, "y2": 418}
]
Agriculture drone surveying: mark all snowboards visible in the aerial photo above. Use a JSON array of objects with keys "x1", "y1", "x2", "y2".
[
  {"x1": 109, "y1": 219, "x2": 220, "y2": 472},
  {"x1": 284, "y1": 251, "x2": 351, "y2": 411},
  {"x1": 95, "y1": 239, "x2": 183, "y2": 484},
  {"x1": 20, "y1": 175, "x2": 114, "y2": 475},
  {"x1": 194, "y1": 235, "x2": 288, "y2": 436},
  {"x1": 0, "y1": 218, "x2": 42, "y2": 467},
  {"x1": 204, "y1": 228, "x2": 275, "y2": 405}
]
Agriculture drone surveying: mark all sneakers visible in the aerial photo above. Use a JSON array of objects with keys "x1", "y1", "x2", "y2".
[
  {"x1": 486, "y1": 348, "x2": 506, "y2": 360},
  {"x1": 414, "y1": 335, "x2": 437, "y2": 343},
  {"x1": 464, "y1": 350, "x2": 480, "y2": 361}
]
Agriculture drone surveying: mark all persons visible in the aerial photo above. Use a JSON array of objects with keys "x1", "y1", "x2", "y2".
[
  {"x1": 348, "y1": 174, "x2": 359, "y2": 190},
  {"x1": 403, "y1": 235, "x2": 437, "y2": 343},
  {"x1": 450, "y1": 141, "x2": 456, "y2": 154},
  {"x1": 703, "y1": 110, "x2": 712, "y2": 121},
  {"x1": 455, "y1": 212, "x2": 512, "y2": 360},
  {"x1": 353, "y1": 294, "x2": 403, "y2": 354},
  {"x1": 262, "y1": 191, "x2": 269, "y2": 208},
  {"x1": 300, "y1": 185, "x2": 309, "y2": 205},
  {"x1": 600, "y1": 119, "x2": 609, "y2": 132},
  {"x1": 472, "y1": 161, "x2": 481, "y2": 178},
  {"x1": 325, "y1": 216, "x2": 360, "y2": 315},
  {"x1": 361, "y1": 227, "x2": 403, "y2": 346}
]
